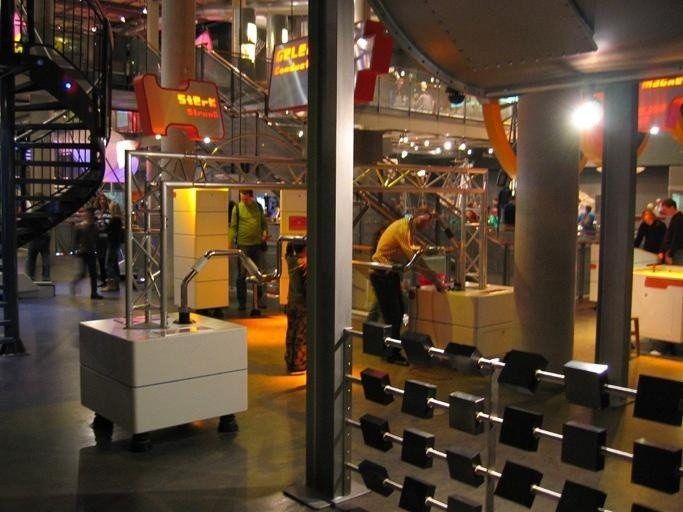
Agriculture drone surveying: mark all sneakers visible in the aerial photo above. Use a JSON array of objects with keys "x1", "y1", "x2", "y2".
[{"x1": 289, "y1": 369, "x2": 306, "y2": 375}]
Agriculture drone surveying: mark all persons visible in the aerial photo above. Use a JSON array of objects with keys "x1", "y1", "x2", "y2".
[
  {"x1": 227, "y1": 189, "x2": 268, "y2": 311},
  {"x1": 413, "y1": 89, "x2": 434, "y2": 111},
  {"x1": 284, "y1": 239, "x2": 307, "y2": 375},
  {"x1": 576, "y1": 205, "x2": 595, "y2": 237},
  {"x1": 634, "y1": 197, "x2": 683, "y2": 266},
  {"x1": 465, "y1": 196, "x2": 516, "y2": 224},
  {"x1": 367, "y1": 213, "x2": 451, "y2": 367},
  {"x1": 25, "y1": 191, "x2": 123, "y2": 300}
]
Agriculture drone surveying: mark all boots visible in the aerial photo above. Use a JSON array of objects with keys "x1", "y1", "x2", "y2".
[{"x1": 100, "y1": 279, "x2": 119, "y2": 291}]
